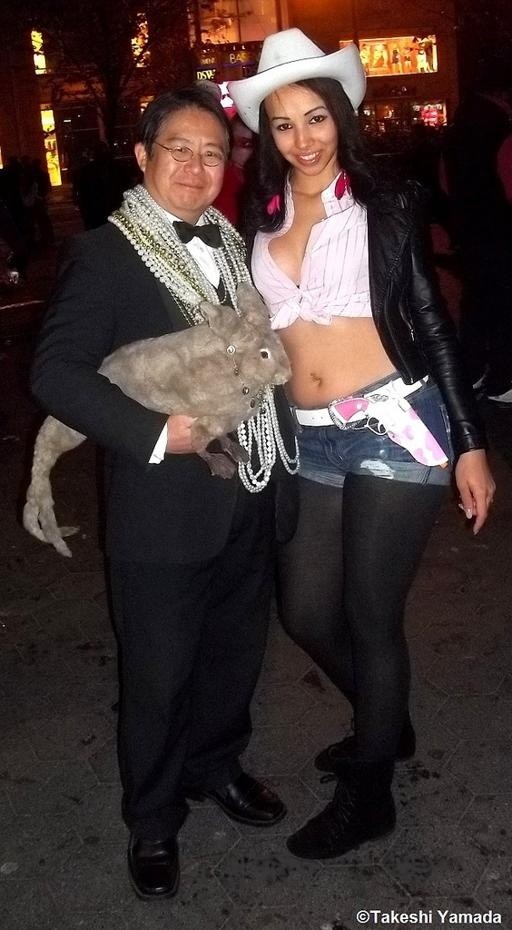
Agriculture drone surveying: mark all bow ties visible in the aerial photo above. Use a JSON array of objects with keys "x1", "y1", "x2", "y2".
[{"x1": 173, "y1": 219, "x2": 222, "y2": 250}]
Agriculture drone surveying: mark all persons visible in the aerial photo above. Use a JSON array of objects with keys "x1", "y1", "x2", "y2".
[
  {"x1": 0, "y1": 155, "x2": 42, "y2": 269},
  {"x1": 218, "y1": 113, "x2": 261, "y2": 225},
  {"x1": 223, "y1": 25, "x2": 498, "y2": 860},
  {"x1": 75, "y1": 139, "x2": 126, "y2": 228},
  {"x1": 436, "y1": 76, "x2": 511, "y2": 405},
  {"x1": 27, "y1": 82, "x2": 287, "y2": 904}
]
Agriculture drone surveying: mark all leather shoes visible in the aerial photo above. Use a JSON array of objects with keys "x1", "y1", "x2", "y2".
[
  {"x1": 183, "y1": 765, "x2": 287, "y2": 826},
  {"x1": 127, "y1": 802, "x2": 191, "y2": 901}
]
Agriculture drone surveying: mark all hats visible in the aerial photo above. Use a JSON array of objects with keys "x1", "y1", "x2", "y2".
[{"x1": 222, "y1": 27, "x2": 367, "y2": 136}]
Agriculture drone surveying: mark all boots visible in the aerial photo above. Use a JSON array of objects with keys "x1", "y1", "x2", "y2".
[
  {"x1": 287, "y1": 745, "x2": 398, "y2": 861},
  {"x1": 314, "y1": 713, "x2": 417, "y2": 771}
]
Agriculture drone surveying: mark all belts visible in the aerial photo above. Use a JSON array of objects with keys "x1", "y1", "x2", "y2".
[{"x1": 289, "y1": 370, "x2": 429, "y2": 431}]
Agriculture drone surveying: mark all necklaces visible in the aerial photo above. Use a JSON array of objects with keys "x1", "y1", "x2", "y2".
[{"x1": 107, "y1": 186, "x2": 295, "y2": 490}]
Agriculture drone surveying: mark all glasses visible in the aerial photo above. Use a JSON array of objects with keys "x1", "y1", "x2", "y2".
[{"x1": 146, "y1": 133, "x2": 229, "y2": 168}]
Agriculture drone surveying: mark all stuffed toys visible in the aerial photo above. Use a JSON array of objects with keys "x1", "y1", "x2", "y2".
[{"x1": 17, "y1": 284, "x2": 292, "y2": 561}]
